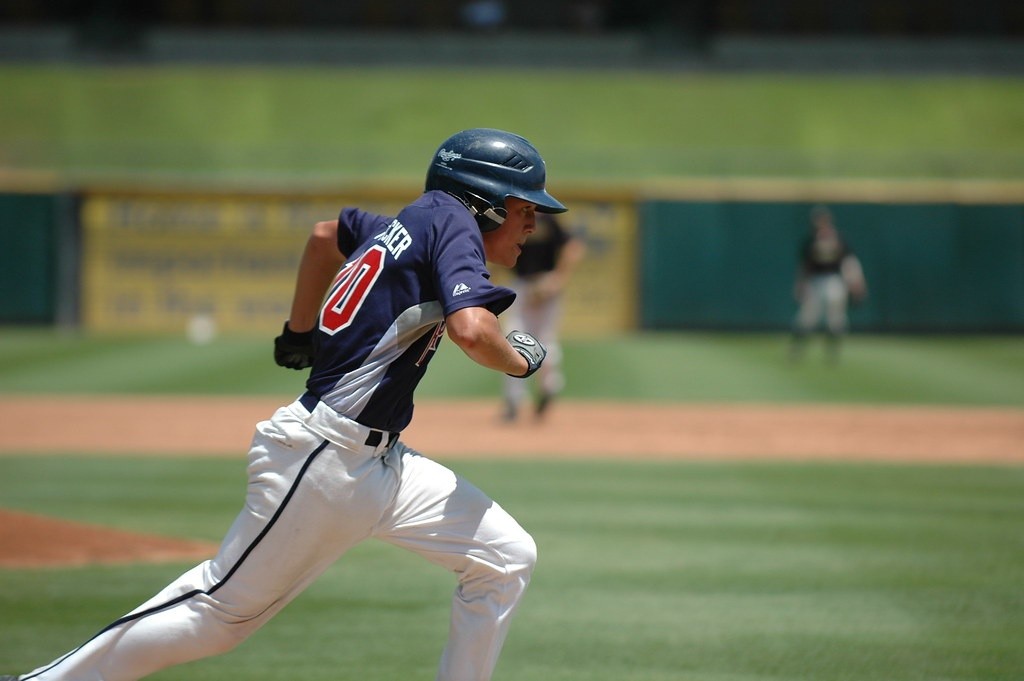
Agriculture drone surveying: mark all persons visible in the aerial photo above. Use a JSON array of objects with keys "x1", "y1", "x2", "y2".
[
  {"x1": 794, "y1": 210, "x2": 868, "y2": 368},
  {"x1": 499, "y1": 212, "x2": 587, "y2": 427},
  {"x1": 0, "y1": 128, "x2": 569, "y2": 681}
]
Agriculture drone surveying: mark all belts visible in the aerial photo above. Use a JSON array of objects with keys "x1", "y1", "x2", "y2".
[{"x1": 300, "y1": 391, "x2": 400, "y2": 450}]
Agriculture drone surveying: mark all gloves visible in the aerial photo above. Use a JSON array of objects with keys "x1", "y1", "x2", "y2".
[
  {"x1": 274, "y1": 316, "x2": 322, "y2": 370},
  {"x1": 505, "y1": 330, "x2": 547, "y2": 378}
]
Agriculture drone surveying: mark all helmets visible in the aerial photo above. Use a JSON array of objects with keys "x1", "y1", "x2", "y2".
[{"x1": 425, "y1": 128, "x2": 568, "y2": 233}]
